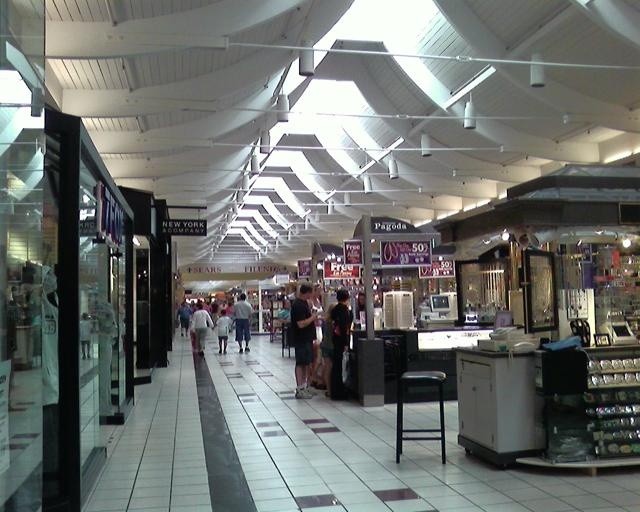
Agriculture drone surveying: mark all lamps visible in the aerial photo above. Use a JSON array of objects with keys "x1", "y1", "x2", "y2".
[
  {"x1": 344, "y1": 189, "x2": 351, "y2": 207},
  {"x1": 327, "y1": 196, "x2": 334, "y2": 217},
  {"x1": 389, "y1": 150, "x2": 399, "y2": 180},
  {"x1": 251, "y1": 145, "x2": 259, "y2": 175},
  {"x1": 463, "y1": 92, "x2": 477, "y2": 129},
  {"x1": 277, "y1": 85, "x2": 289, "y2": 123},
  {"x1": 298, "y1": 34, "x2": 315, "y2": 76},
  {"x1": 421, "y1": 127, "x2": 431, "y2": 156},
  {"x1": 363, "y1": 154, "x2": 372, "y2": 196},
  {"x1": 207, "y1": 175, "x2": 249, "y2": 264},
  {"x1": 260, "y1": 113, "x2": 271, "y2": 155},
  {"x1": 529, "y1": 33, "x2": 546, "y2": 86},
  {"x1": 254, "y1": 214, "x2": 309, "y2": 262}
]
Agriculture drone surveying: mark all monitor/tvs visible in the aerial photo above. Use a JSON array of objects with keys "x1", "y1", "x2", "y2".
[
  {"x1": 612, "y1": 325, "x2": 631, "y2": 336},
  {"x1": 431, "y1": 294, "x2": 452, "y2": 318}
]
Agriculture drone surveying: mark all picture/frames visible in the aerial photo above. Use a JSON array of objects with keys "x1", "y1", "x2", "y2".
[
  {"x1": 520, "y1": 248, "x2": 561, "y2": 335},
  {"x1": 593, "y1": 332, "x2": 611, "y2": 347},
  {"x1": 454, "y1": 256, "x2": 511, "y2": 327}
]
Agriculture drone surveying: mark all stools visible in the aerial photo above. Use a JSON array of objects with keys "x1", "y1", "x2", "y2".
[{"x1": 394, "y1": 368, "x2": 453, "y2": 467}]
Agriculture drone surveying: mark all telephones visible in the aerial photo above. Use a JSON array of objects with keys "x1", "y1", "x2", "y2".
[{"x1": 513, "y1": 342, "x2": 536, "y2": 352}]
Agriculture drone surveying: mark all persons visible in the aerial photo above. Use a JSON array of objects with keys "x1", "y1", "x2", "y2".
[{"x1": 175, "y1": 283, "x2": 367, "y2": 399}]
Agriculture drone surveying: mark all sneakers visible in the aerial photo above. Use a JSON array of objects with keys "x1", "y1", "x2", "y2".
[
  {"x1": 295, "y1": 386, "x2": 314, "y2": 399},
  {"x1": 245, "y1": 348, "x2": 251, "y2": 352},
  {"x1": 238, "y1": 349, "x2": 243, "y2": 353}
]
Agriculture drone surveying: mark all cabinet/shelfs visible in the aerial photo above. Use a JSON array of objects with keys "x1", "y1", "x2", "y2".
[
  {"x1": 452, "y1": 345, "x2": 548, "y2": 453},
  {"x1": 536, "y1": 340, "x2": 640, "y2": 462}
]
